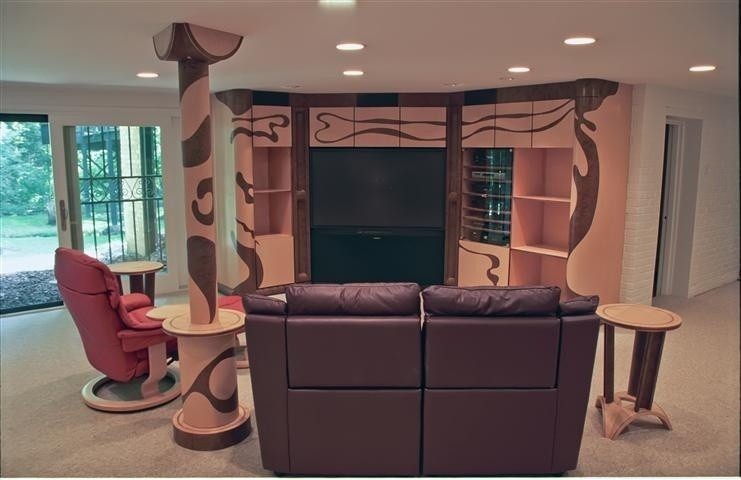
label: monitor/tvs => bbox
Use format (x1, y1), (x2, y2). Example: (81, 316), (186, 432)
(309, 147), (446, 230)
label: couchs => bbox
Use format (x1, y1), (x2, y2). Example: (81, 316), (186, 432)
(243, 282), (604, 477)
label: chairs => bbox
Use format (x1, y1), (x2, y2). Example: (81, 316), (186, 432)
(54, 247), (182, 413)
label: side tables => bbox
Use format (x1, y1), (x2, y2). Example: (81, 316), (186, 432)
(106, 259), (165, 307)
(596, 303), (683, 440)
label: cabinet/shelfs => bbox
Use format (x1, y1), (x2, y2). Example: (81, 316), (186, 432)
(208, 89), (298, 298)
(458, 78), (634, 306)
